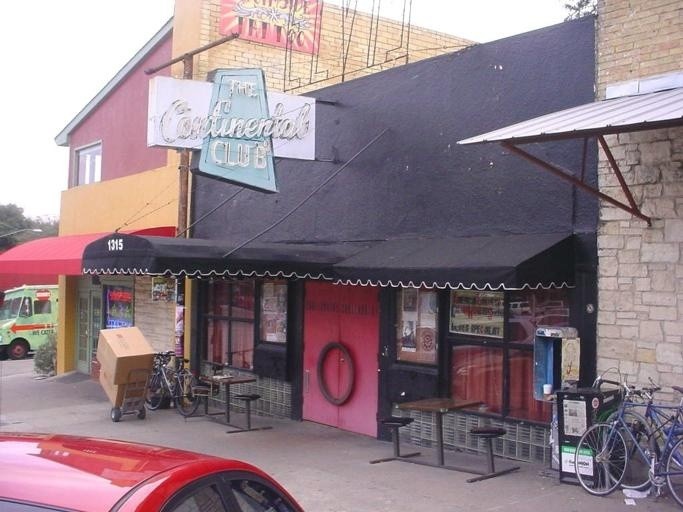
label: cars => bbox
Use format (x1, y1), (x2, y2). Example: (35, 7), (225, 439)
(0, 431), (304, 511)
(454, 323), (570, 409)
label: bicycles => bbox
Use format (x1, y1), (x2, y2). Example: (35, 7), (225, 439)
(574, 367), (683, 505)
(145, 351), (201, 416)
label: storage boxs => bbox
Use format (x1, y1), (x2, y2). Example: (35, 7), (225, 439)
(98, 365), (149, 408)
(95, 327), (156, 385)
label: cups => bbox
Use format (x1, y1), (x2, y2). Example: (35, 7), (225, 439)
(543, 383), (552, 401)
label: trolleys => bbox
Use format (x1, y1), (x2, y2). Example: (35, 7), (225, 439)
(111, 369), (152, 422)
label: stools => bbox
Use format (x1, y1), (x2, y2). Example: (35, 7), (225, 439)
(185, 386), (227, 418)
(227, 392), (273, 433)
(368, 415), (422, 463)
(467, 425), (520, 484)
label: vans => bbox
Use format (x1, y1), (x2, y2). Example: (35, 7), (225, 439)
(507, 300), (570, 347)
(0, 285), (59, 360)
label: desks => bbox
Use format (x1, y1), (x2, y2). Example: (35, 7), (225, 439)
(198, 372), (257, 430)
(395, 396), (487, 476)
